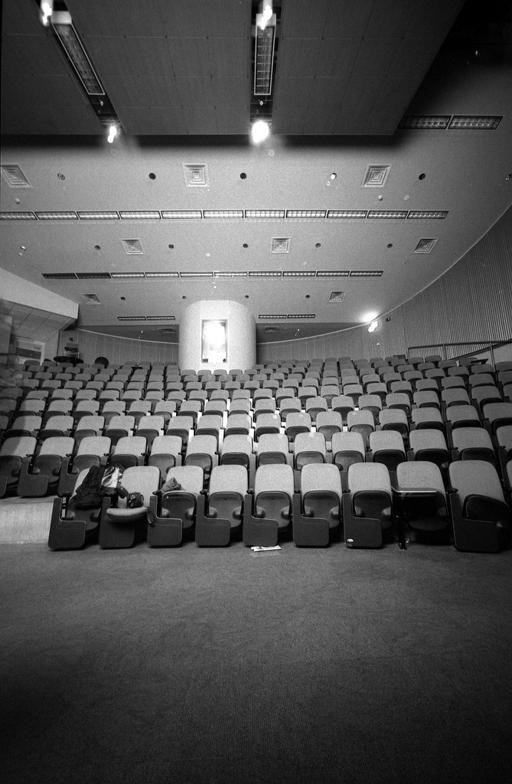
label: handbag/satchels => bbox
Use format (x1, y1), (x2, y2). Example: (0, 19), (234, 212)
(126, 493), (145, 508)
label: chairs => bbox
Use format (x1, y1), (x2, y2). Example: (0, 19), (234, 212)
(0, 355), (511, 553)
